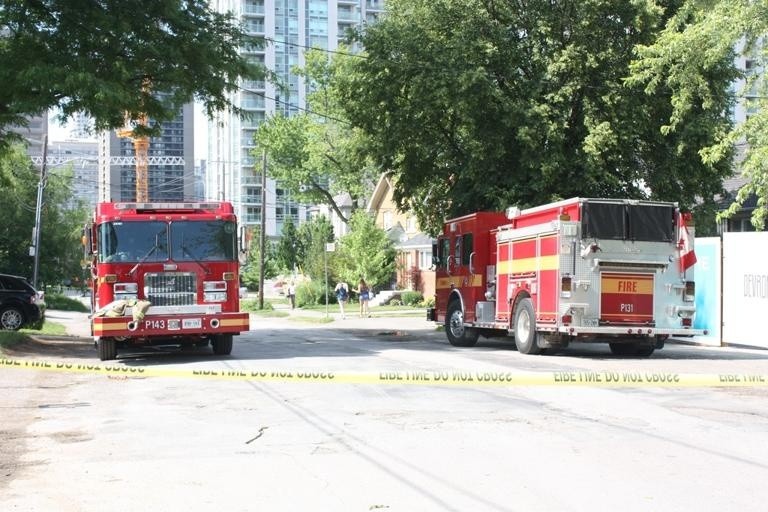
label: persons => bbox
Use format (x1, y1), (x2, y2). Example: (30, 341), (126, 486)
(351, 278), (372, 318)
(286, 280), (296, 309)
(335, 278), (350, 319)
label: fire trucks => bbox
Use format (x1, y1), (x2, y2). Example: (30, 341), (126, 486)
(79, 197), (251, 364)
(425, 192), (711, 358)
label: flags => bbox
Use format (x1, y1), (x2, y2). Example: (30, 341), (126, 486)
(677, 212), (697, 273)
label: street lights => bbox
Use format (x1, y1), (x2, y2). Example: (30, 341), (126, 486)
(32, 156), (100, 288)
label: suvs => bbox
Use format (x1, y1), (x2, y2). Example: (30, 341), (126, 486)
(0, 272), (47, 332)
